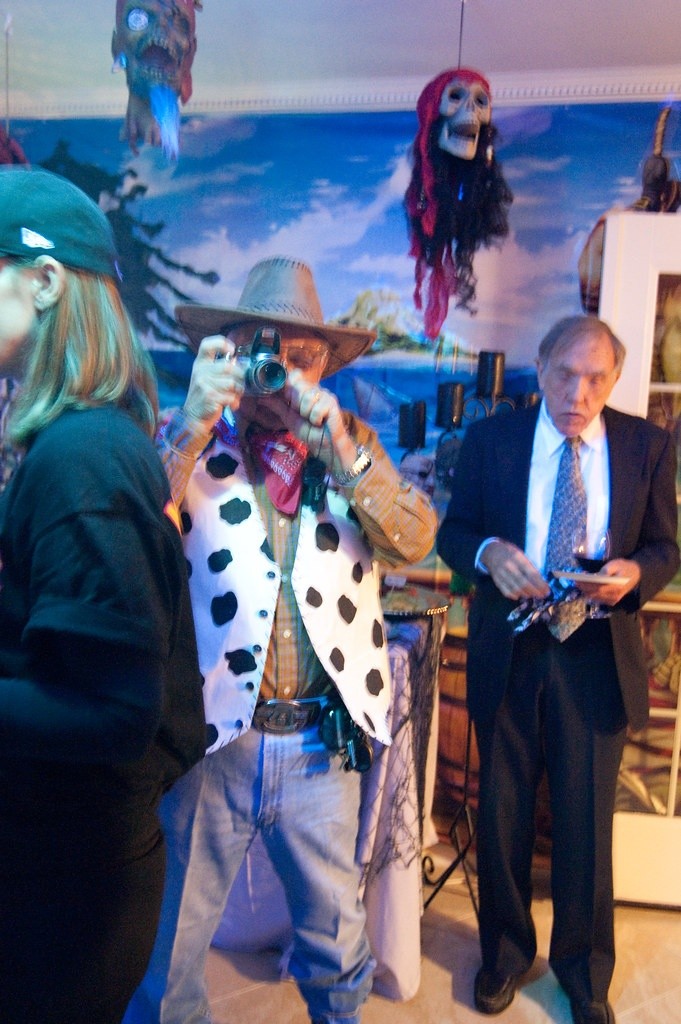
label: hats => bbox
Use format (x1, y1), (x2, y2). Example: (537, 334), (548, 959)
(173, 256), (377, 381)
(0, 166), (119, 277)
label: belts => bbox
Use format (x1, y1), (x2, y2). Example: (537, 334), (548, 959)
(252, 694), (339, 736)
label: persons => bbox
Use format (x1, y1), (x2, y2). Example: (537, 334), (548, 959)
(440, 314), (681, 1024)
(0, 167), (207, 1024)
(121, 259), (438, 1024)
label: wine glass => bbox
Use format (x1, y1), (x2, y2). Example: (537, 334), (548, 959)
(573, 526), (613, 619)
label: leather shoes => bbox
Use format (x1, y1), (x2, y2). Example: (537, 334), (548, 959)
(473, 965), (517, 1014)
(569, 996), (616, 1024)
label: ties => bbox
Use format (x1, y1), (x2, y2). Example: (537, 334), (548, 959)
(542, 438), (591, 644)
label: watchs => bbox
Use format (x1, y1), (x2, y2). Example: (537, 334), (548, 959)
(335, 446), (372, 485)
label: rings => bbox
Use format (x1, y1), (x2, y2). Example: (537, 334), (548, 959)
(312, 393), (318, 401)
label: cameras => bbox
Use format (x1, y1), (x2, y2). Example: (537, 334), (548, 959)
(214, 325), (286, 399)
